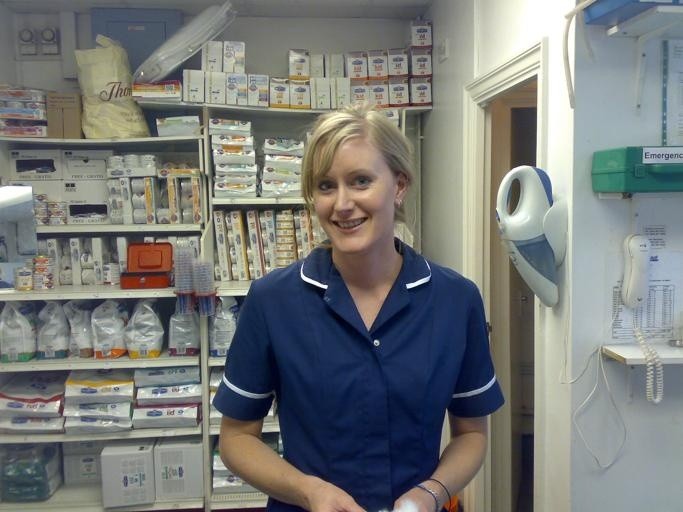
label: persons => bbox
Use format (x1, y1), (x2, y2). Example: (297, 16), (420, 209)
(211, 107), (508, 511)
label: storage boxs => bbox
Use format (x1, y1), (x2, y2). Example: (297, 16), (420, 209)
(46, 92), (83, 139)
(181, 21), (433, 110)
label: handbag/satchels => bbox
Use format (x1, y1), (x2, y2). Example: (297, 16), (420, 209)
(132, 1), (236, 83)
(74, 34), (151, 139)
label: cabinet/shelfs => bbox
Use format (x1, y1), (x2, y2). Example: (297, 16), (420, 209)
(598, 7), (682, 402)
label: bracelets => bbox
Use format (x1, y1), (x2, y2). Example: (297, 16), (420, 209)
(414, 483), (442, 512)
(423, 477), (452, 510)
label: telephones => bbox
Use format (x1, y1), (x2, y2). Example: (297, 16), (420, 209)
(622, 233), (652, 308)
